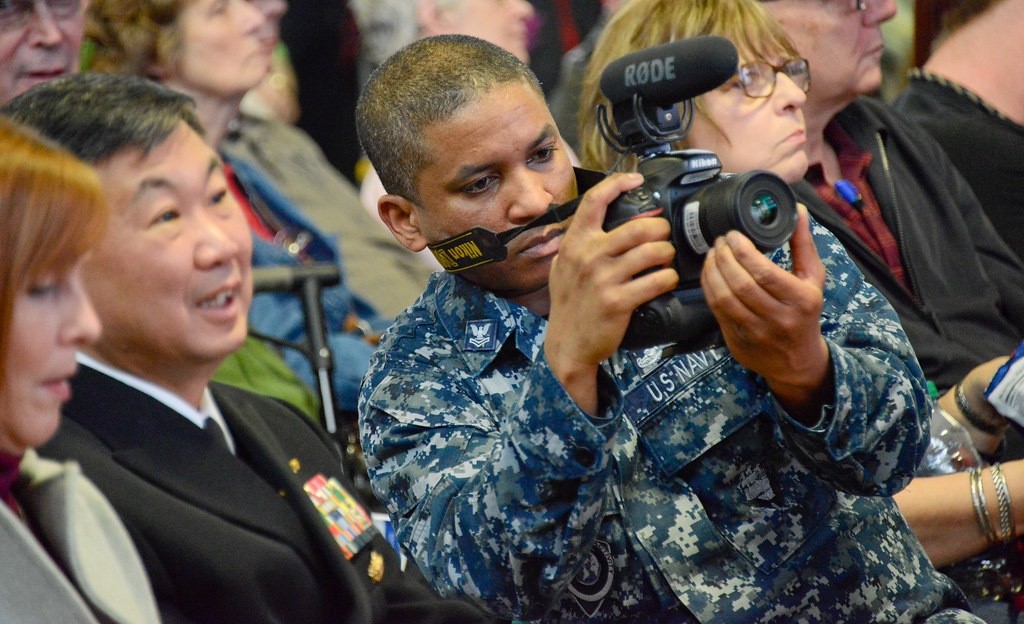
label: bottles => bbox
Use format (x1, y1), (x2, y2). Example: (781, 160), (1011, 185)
(914, 380), (983, 477)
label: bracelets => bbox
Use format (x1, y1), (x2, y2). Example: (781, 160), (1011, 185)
(970, 462), (1016, 546)
(955, 382), (1010, 436)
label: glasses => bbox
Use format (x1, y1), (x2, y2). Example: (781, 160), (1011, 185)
(717, 57), (811, 99)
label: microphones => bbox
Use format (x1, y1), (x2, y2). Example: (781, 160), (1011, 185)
(600, 36), (737, 111)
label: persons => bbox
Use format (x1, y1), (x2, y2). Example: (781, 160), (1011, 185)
(0, 0), (90, 101)
(0, 71), (490, 624)
(82, 0), (450, 419)
(0, 117), (162, 624)
(754, 0), (1024, 459)
(354, 33), (984, 624)
(582, 1), (1024, 624)
(892, 0), (1024, 264)
(360, 0), (534, 267)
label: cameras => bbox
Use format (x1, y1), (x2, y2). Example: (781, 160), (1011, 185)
(601, 147), (798, 350)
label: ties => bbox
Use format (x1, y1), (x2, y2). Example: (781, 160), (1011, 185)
(204, 418), (231, 455)
